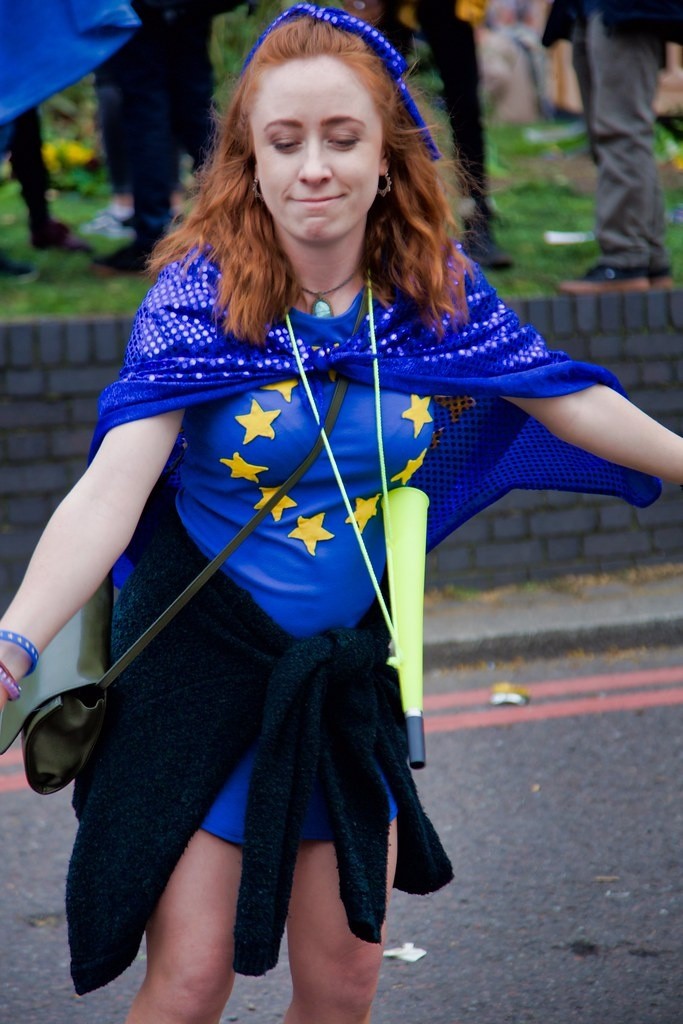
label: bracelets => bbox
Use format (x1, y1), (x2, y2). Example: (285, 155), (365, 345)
(0, 630), (38, 676)
(0, 665), (23, 701)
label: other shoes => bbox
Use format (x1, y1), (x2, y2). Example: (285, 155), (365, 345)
(78, 202), (137, 240)
(32, 226), (95, 255)
(649, 267), (674, 291)
(168, 207), (186, 232)
(0, 252), (34, 284)
(462, 216), (512, 269)
(88, 226), (166, 275)
(557, 264), (649, 293)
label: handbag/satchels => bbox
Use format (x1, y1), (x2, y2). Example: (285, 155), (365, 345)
(0, 578), (108, 796)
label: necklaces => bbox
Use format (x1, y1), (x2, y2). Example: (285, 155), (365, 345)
(301, 272), (357, 318)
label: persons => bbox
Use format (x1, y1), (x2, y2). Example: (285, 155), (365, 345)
(83, 0), (261, 271)
(542, 0), (683, 292)
(344, 1), (515, 272)
(483, 1), (585, 121)
(0, 0), (682, 1024)
(0, 0), (141, 279)
